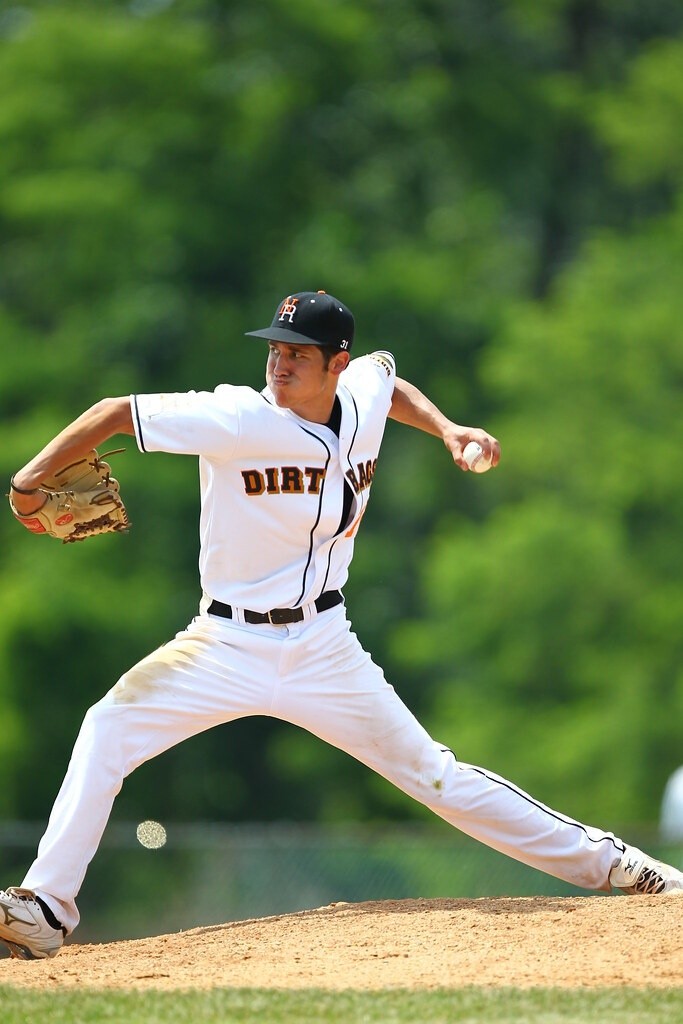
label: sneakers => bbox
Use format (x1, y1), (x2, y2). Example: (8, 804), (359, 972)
(609, 841), (683, 894)
(0, 887), (67, 960)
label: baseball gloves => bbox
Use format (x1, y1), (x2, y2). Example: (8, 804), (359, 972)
(12, 451), (129, 544)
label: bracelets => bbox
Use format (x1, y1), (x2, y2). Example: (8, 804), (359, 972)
(10, 474), (37, 495)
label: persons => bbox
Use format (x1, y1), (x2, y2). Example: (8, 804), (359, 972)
(0, 289), (683, 960)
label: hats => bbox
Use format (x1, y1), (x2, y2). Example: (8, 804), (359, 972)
(243, 289), (354, 351)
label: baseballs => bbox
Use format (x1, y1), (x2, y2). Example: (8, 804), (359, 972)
(464, 443), (494, 473)
(139, 822), (167, 849)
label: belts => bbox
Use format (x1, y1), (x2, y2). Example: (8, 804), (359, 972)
(207, 590), (343, 627)
